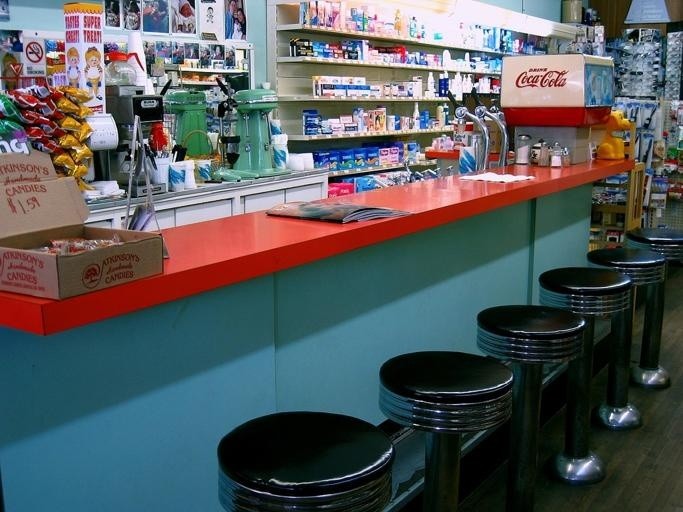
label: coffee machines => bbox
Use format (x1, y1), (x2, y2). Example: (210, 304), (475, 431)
(213, 79), (292, 183)
(163, 90), (211, 158)
(105, 79), (174, 198)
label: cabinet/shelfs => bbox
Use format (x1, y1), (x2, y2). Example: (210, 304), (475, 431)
(84, 164), (333, 233)
(590, 162), (648, 244)
(265, 0), (587, 188)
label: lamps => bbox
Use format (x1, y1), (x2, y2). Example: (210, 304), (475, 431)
(451, 1), (553, 39)
(624, 0), (672, 24)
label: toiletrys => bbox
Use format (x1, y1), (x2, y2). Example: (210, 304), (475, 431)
(421, 25), (425, 38)
(458, 21), (500, 53)
(417, 31), (421, 39)
(434, 33), (443, 40)
(298, 1), (341, 30)
(394, 10), (402, 35)
(347, 6), (394, 34)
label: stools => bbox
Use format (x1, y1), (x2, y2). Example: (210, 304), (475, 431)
(538, 267), (632, 485)
(476, 305), (586, 512)
(379, 350), (514, 511)
(217, 410), (395, 510)
(586, 246), (667, 432)
(624, 228), (682, 389)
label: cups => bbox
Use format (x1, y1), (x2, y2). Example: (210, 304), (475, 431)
(151, 158), (168, 192)
(197, 160), (211, 181)
(169, 163), (185, 192)
(273, 143), (287, 171)
(182, 160), (196, 189)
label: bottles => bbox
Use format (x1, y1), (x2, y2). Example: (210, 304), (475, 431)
(514, 134), (531, 164)
(442, 103), (449, 125)
(550, 141), (563, 168)
(563, 147), (570, 167)
(444, 72), (449, 96)
(439, 74), (444, 96)
(439, 111), (445, 126)
(428, 72), (434, 97)
(413, 102), (420, 119)
(538, 142), (550, 166)
(436, 103), (443, 120)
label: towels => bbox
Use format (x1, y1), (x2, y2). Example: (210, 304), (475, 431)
(458, 172), (538, 182)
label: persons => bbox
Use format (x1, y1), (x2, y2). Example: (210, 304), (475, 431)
(173, 0), (196, 35)
(143, 40), (250, 69)
(224, 0), (246, 41)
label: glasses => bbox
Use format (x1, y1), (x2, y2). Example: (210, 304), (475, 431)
(630, 72), (643, 75)
(665, 33), (682, 101)
(615, 49), (633, 57)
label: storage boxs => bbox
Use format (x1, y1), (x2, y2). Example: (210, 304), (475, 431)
(0, 151), (164, 300)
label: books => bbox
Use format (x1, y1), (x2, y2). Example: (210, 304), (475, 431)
(588, 231), (603, 252)
(265, 200), (410, 223)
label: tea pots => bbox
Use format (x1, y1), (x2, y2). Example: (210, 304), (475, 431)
(104, 51), (144, 86)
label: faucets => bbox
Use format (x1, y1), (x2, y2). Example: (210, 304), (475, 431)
(476, 105), (509, 168)
(454, 106), (492, 172)
